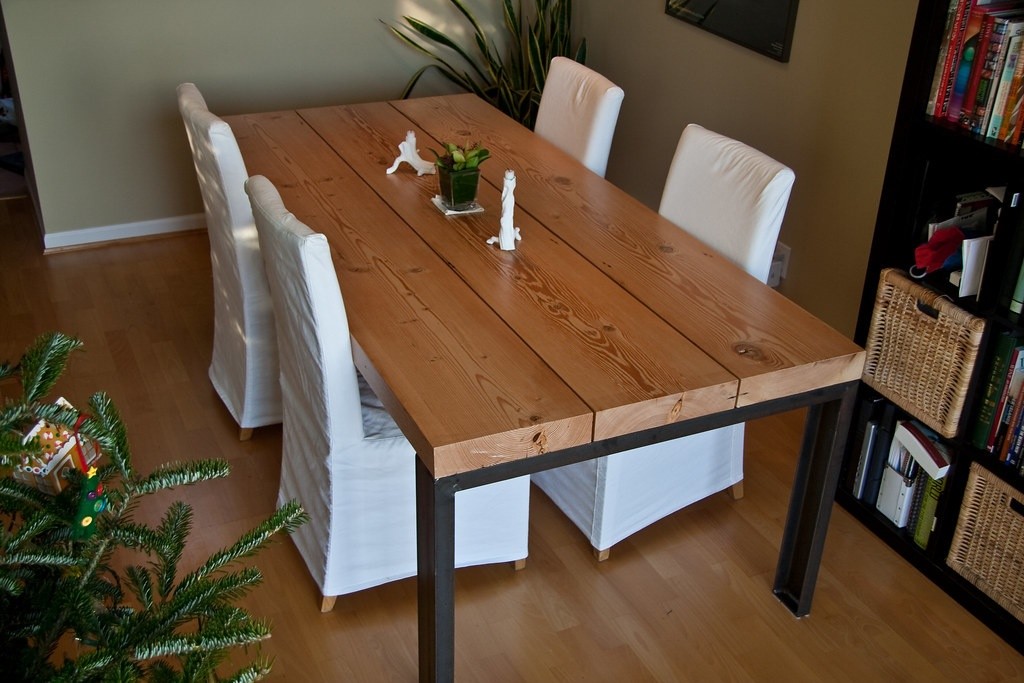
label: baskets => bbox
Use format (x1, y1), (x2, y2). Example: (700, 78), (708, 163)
(945, 462), (1024, 623)
(860, 268), (985, 438)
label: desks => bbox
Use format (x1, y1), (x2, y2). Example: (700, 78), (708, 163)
(218, 93), (866, 683)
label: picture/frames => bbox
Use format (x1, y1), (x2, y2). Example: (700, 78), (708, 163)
(665, 0), (800, 63)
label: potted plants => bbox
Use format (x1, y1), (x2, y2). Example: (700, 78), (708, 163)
(426, 138), (492, 212)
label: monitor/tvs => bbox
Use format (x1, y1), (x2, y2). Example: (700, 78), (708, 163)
(665, 0), (800, 63)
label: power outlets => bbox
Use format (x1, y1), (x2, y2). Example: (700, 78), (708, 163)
(775, 241), (792, 279)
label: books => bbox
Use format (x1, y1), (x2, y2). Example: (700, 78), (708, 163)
(1010, 261), (1024, 313)
(886, 418), (952, 550)
(926, 0), (1024, 149)
(953, 197), (993, 217)
(972, 332), (1024, 477)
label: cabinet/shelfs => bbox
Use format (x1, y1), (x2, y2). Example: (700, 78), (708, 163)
(834, 0), (1024, 655)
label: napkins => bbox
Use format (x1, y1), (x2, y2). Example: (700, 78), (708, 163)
(430, 195), (485, 216)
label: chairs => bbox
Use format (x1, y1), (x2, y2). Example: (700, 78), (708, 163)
(531, 123), (796, 563)
(534, 56), (625, 179)
(243, 173), (530, 612)
(176, 82), (283, 441)
